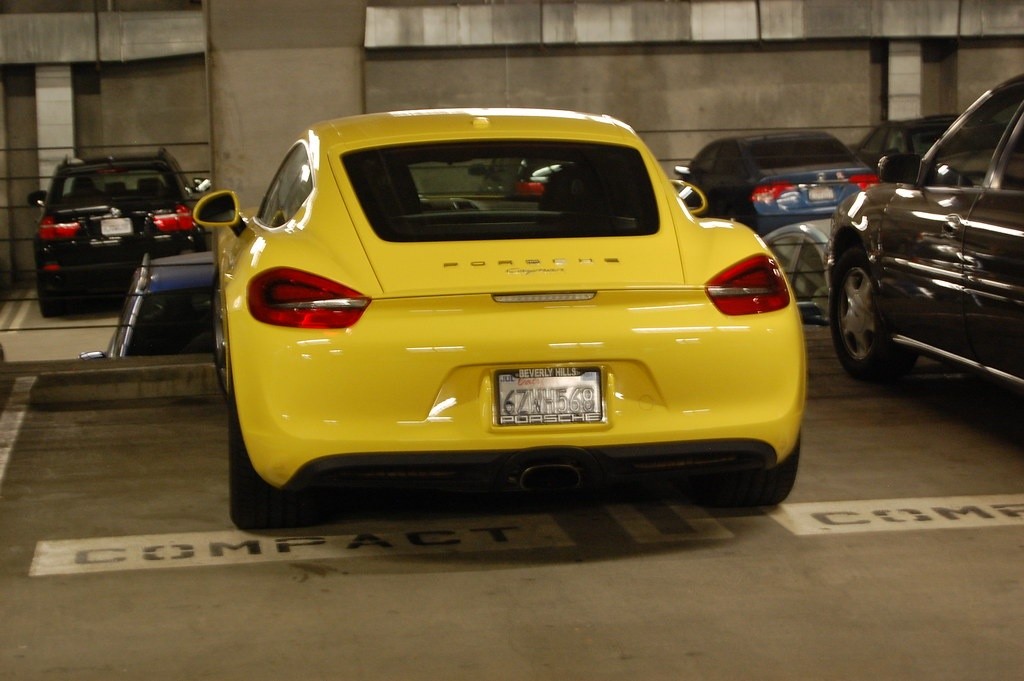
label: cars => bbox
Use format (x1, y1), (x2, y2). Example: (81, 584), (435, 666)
(673, 130), (883, 238)
(851, 115), (960, 175)
(193, 106), (806, 532)
(828, 74), (1024, 399)
(762, 219), (834, 323)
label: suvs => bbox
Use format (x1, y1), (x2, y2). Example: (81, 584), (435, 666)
(77, 251), (217, 358)
(27, 148), (210, 315)
